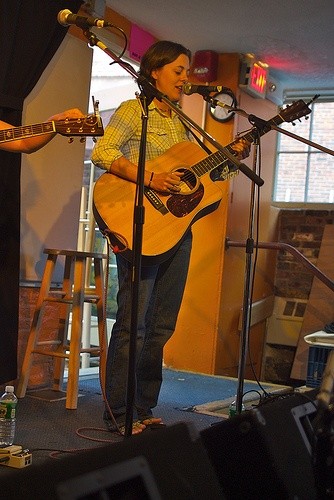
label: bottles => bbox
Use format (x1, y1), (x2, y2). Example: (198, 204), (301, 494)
(0, 386), (17, 445)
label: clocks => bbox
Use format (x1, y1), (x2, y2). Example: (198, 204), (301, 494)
(208, 88), (236, 122)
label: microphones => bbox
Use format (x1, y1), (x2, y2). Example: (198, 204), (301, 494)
(183, 83), (226, 96)
(57, 9), (112, 29)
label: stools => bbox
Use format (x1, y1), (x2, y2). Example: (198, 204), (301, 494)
(13, 248), (110, 410)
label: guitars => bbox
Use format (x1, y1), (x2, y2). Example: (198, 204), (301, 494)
(0, 96), (105, 144)
(92, 98), (313, 266)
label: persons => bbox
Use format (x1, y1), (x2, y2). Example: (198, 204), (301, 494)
(90, 40), (251, 438)
(0, 107), (86, 154)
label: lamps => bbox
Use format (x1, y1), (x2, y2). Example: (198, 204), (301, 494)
(266, 83), (277, 92)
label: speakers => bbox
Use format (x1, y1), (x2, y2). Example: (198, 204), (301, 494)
(0, 421), (226, 500)
(200, 387), (334, 500)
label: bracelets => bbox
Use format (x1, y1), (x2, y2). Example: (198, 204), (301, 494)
(147, 171), (154, 188)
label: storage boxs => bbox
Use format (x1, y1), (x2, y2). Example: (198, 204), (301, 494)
(306, 345), (334, 388)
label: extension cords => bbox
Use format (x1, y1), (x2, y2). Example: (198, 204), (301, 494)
(0, 445), (33, 469)
